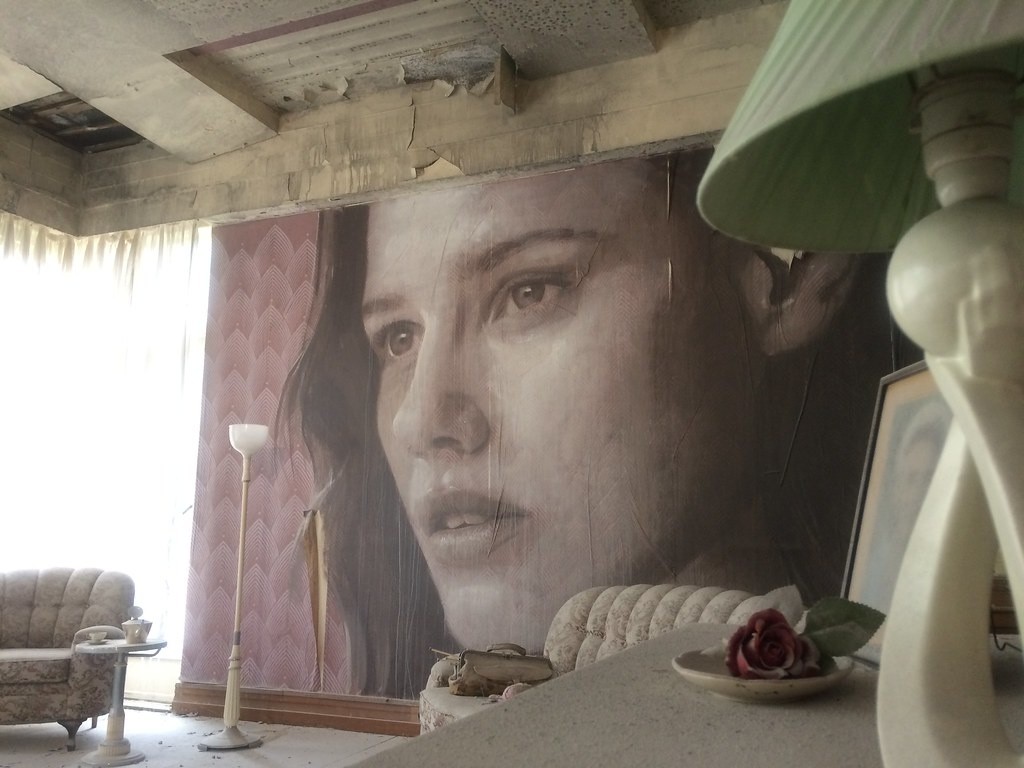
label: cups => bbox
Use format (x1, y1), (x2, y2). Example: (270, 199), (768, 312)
(86, 632), (106, 641)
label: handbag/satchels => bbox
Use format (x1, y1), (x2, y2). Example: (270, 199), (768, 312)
(448, 642), (553, 697)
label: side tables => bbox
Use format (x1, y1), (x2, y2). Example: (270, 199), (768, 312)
(74, 637), (168, 766)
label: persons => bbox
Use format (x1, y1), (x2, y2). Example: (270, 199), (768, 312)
(274, 143), (929, 702)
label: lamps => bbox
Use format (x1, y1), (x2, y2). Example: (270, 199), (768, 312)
(197, 423), (271, 751)
(693, 0), (1021, 768)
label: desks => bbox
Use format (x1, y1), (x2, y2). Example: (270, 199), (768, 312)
(344, 623), (1021, 768)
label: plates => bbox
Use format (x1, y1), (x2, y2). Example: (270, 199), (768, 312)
(87, 640), (108, 644)
(670, 646), (857, 703)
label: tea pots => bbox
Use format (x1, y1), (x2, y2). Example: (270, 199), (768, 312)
(121, 606), (154, 643)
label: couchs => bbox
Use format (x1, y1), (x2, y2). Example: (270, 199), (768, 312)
(417, 584), (763, 737)
(0, 569), (133, 750)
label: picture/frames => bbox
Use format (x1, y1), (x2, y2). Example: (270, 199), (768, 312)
(837, 359), (954, 671)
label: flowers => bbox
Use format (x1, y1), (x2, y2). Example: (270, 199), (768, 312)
(722, 596), (887, 681)
